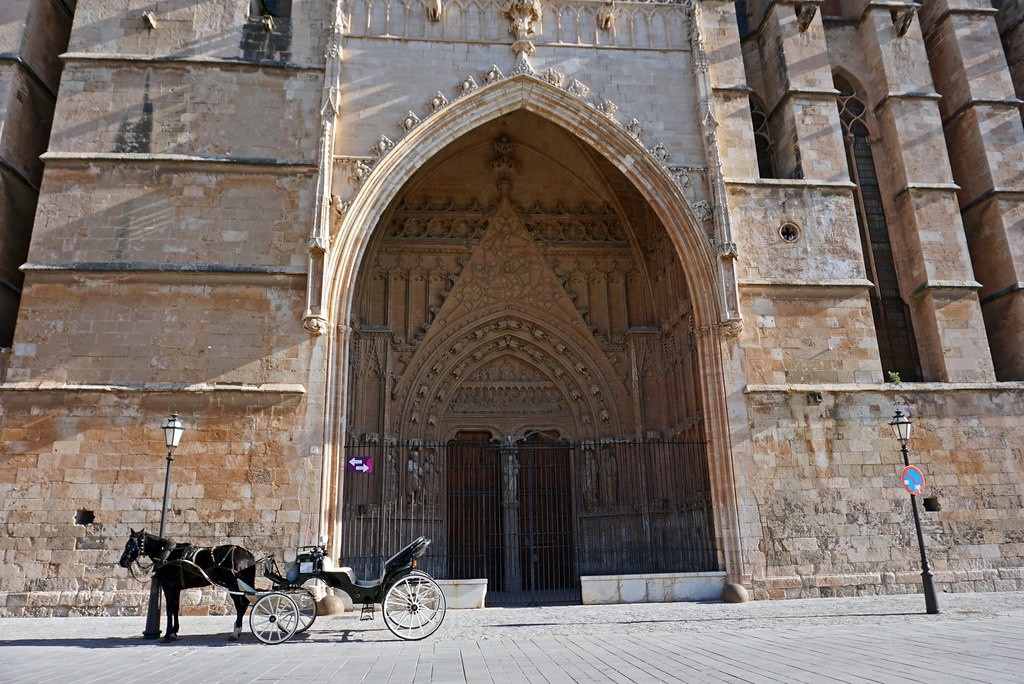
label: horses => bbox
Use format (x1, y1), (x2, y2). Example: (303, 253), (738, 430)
(121, 527), (256, 642)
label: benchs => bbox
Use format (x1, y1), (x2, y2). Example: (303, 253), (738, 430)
(380, 556), (405, 581)
(323, 556), (357, 586)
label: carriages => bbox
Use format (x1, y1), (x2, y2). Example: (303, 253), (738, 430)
(118, 527), (447, 645)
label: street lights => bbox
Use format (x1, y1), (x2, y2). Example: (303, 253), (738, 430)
(141, 410), (186, 638)
(887, 408), (941, 616)
